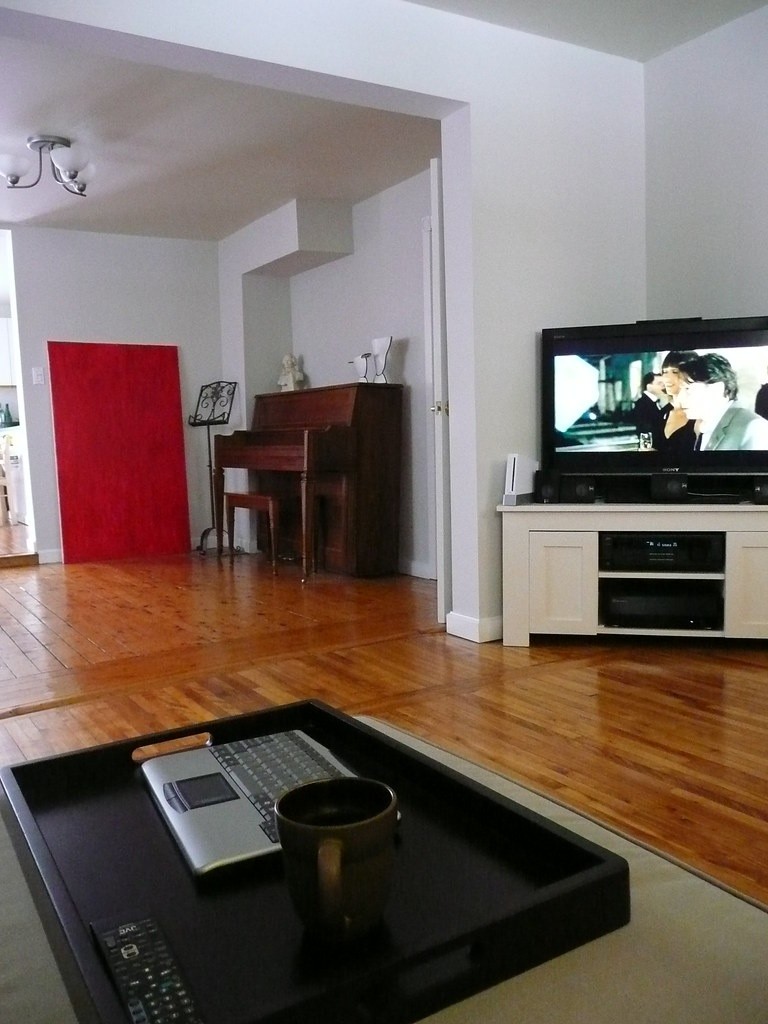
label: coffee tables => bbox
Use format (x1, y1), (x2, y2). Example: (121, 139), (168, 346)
(0, 714), (768, 1024)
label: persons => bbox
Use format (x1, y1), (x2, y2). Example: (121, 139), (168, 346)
(277, 354), (304, 386)
(634, 350), (768, 450)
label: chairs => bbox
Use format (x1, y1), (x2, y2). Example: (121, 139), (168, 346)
(0, 437), (18, 527)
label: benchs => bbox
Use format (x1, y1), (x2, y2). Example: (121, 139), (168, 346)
(220, 490), (325, 573)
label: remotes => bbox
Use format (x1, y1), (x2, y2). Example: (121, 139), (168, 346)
(90, 908), (203, 1024)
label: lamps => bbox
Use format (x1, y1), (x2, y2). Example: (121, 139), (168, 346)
(0, 135), (97, 197)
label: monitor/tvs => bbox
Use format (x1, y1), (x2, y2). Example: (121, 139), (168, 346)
(540, 316), (768, 505)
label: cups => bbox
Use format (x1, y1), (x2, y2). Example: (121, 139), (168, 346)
(640, 432), (652, 450)
(273, 777), (397, 936)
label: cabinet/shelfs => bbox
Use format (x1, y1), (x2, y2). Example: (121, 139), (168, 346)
(497, 502), (768, 648)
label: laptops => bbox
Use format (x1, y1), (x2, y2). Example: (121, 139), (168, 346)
(142, 728), (401, 876)
